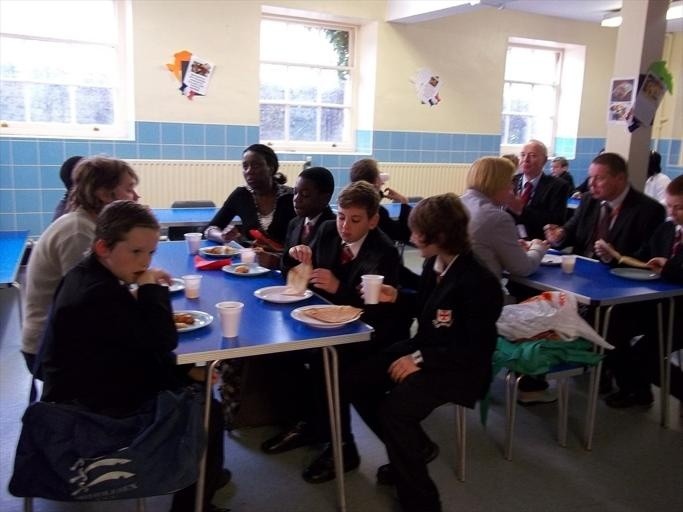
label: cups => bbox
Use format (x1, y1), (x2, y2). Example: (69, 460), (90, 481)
(561, 255), (578, 273)
(361, 274), (384, 305)
(182, 275), (203, 299)
(184, 233), (203, 255)
(215, 301), (244, 338)
(380, 173), (391, 184)
(240, 248), (256, 263)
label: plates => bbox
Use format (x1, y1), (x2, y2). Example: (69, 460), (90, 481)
(540, 253), (563, 266)
(172, 310), (213, 332)
(199, 245), (241, 258)
(157, 278), (186, 292)
(253, 285), (313, 304)
(222, 262), (270, 276)
(291, 304), (361, 328)
(610, 267), (661, 281)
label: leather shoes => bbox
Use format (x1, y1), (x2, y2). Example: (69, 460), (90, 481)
(215, 468), (231, 491)
(602, 388), (655, 410)
(302, 438), (360, 484)
(260, 424), (322, 455)
(205, 505), (232, 512)
(398, 477), (442, 512)
(377, 439), (440, 483)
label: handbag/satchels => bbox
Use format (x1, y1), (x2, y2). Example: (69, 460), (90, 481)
(6, 377), (208, 504)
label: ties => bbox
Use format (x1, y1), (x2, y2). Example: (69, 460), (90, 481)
(339, 243), (356, 266)
(669, 230), (682, 260)
(300, 222), (314, 243)
(520, 182), (532, 212)
(591, 203), (611, 253)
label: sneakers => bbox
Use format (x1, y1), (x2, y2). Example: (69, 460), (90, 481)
(516, 387), (559, 406)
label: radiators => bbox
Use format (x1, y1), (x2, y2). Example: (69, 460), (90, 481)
(120, 158), (304, 209)
(378, 162), (469, 208)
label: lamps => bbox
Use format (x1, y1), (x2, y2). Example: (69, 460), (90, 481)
(599, 0), (683, 28)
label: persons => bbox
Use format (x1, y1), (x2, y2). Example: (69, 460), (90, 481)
(350, 192), (504, 511)
(30, 198), (232, 512)
(572, 148), (603, 198)
(18, 154), (139, 381)
(258, 181), (403, 484)
(502, 143), (569, 241)
(50, 154), (84, 221)
(459, 156), (557, 403)
(350, 160), (414, 246)
(644, 152), (672, 206)
(551, 156), (575, 189)
(543, 154), (664, 393)
(249, 167), (335, 268)
(594, 176), (681, 408)
(202, 143), (292, 247)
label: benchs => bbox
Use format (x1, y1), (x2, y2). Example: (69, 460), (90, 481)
(503, 358), (593, 461)
(0, 233), (35, 328)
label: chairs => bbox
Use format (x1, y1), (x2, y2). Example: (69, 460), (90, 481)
(167, 198), (215, 241)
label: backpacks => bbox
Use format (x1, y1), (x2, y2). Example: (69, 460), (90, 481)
(488, 333), (601, 379)
